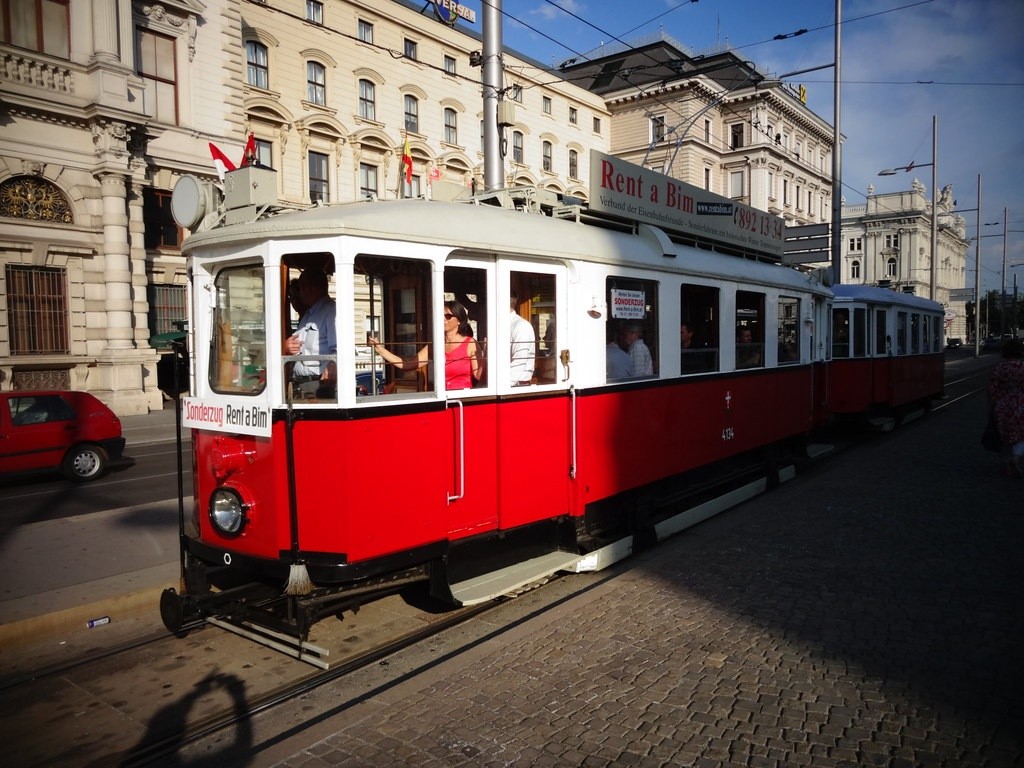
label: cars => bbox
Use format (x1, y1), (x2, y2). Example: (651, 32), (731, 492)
(947, 338), (963, 349)
(970, 333), (1018, 352)
(0, 388), (125, 483)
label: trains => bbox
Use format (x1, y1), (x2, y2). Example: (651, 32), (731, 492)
(159, 147), (945, 670)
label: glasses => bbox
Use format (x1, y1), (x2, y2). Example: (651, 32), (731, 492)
(444, 313), (456, 320)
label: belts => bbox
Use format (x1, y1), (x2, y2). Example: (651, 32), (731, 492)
(291, 375), (322, 384)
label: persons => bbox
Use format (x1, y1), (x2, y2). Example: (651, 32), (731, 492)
(366, 296), (487, 390)
(507, 285), (539, 390)
(287, 274), (308, 322)
(285, 267), (339, 401)
(986, 337), (1023, 476)
(604, 305), (764, 380)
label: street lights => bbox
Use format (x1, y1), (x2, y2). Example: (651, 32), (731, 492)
(877, 115), (938, 301)
(938, 174), (979, 358)
(756, 0), (841, 285)
(972, 207), (1008, 342)
